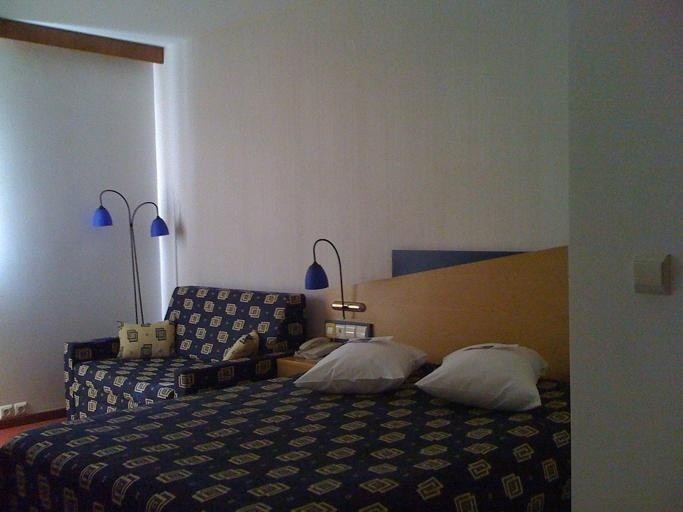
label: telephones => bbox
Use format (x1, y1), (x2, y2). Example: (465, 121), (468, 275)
(297, 336), (342, 360)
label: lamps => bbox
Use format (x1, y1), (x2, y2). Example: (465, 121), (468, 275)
(303, 238), (348, 319)
(92, 189), (170, 325)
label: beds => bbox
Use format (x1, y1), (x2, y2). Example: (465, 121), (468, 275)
(2, 246), (570, 512)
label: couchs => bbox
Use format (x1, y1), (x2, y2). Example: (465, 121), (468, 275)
(60, 283), (307, 424)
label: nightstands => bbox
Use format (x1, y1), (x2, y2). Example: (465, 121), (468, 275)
(274, 353), (318, 379)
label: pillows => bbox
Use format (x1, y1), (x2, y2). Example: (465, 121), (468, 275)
(116, 316), (178, 362)
(218, 328), (261, 363)
(294, 335), (552, 412)
